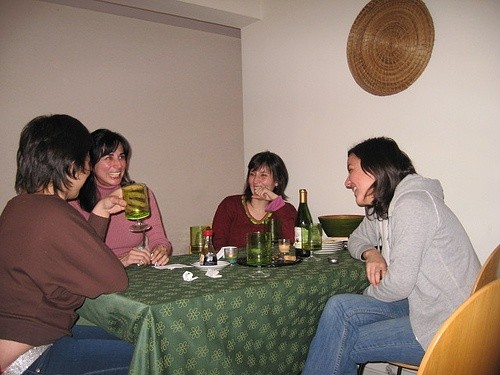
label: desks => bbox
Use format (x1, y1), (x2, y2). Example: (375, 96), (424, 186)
(75, 247), (371, 375)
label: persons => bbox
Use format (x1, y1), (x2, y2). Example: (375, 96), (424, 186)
(300, 136), (482, 375)
(212, 152), (297, 253)
(67, 129), (172, 265)
(0, 114), (145, 374)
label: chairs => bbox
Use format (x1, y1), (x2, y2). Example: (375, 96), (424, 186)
(358, 244), (500, 375)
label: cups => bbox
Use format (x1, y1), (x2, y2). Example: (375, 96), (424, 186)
(190, 226), (210, 252)
(222, 246), (238, 263)
(278, 239), (290, 255)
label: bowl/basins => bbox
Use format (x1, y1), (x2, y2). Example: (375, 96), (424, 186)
(318, 215), (365, 238)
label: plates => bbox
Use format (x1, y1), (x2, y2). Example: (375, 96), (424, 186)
(236, 254), (303, 267)
(313, 237), (348, 254)
(192, 261), (230, 271)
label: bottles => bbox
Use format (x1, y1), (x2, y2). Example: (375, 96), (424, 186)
(294, 189), (313, 257)
(200, 230), (217, 266)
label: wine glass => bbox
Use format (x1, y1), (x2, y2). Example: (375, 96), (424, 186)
(264, 218), (282, 248)
(122, 183), (151, 232)
(301, 223), (322, 262)
(246, 232), (272, 278)
